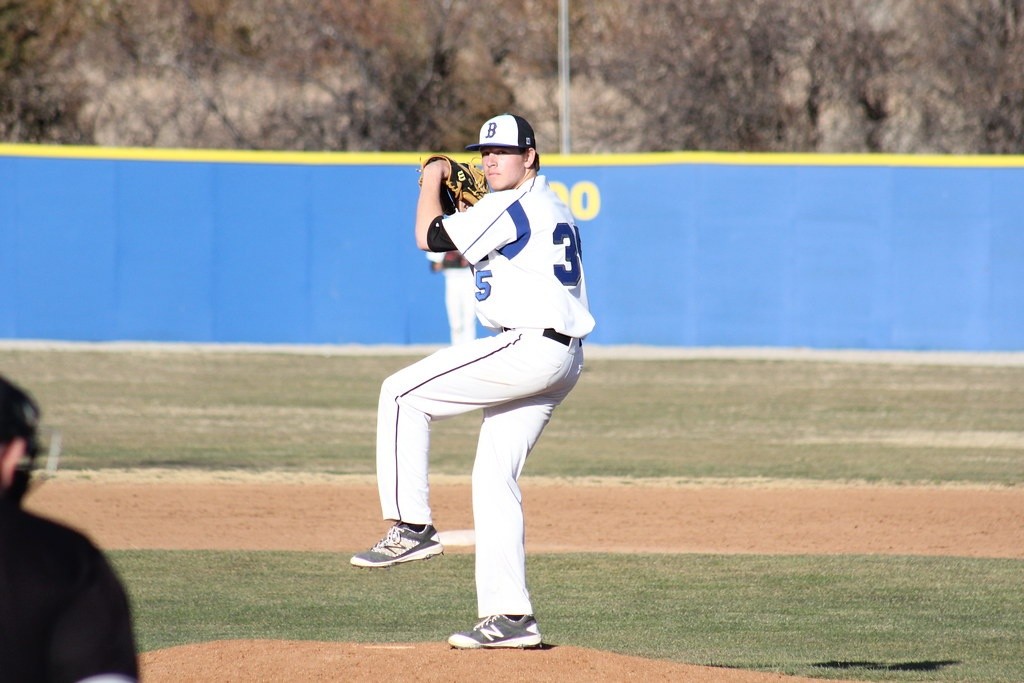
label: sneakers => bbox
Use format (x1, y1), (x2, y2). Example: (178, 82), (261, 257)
(448, 614), (541, 649)
(350, 524), (443, 568)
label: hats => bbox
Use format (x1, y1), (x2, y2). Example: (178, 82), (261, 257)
(465, 112), (536, 151)
(0, 375), (48, 457)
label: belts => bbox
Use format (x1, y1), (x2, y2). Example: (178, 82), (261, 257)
(501, 328), (583, 347)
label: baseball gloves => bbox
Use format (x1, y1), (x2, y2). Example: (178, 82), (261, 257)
(416, 155), (492, 216)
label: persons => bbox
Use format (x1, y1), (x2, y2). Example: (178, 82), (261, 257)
(350, 112), (597, 651)
(0, 374), (139, 683)
